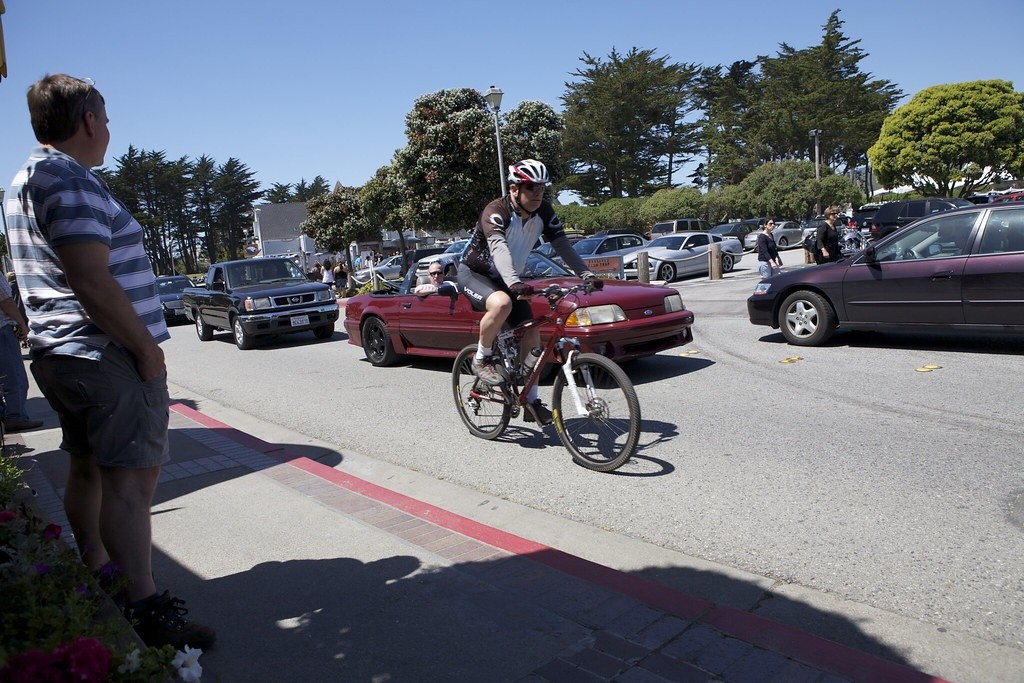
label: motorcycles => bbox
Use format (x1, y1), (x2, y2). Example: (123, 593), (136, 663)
(837, 220), (874, 260)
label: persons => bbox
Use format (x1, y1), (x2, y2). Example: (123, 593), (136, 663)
(271, 265), (281, 277)
(0, 270), (44, 433)
(311, 252), (383, 298)
(415, 262), (458, 296)
(457, 159), (605, 425)
(907, 227), (971, 259)
(758, 218), (783, 277)
(815, 206), (843, 264)
(7, 73), (217, 653)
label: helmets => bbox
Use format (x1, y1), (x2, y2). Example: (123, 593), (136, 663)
(507, 159), (549, 183)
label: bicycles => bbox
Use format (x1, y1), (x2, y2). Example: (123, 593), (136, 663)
(449, 269), (642, 475)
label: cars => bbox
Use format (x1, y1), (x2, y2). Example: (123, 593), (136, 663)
(589, 228), (652, 241)
(745, 217), (767, 228)
(154, 275), (200, 326)
(744, 220), (803, 250)
(708, 222), (750, 248)
(352, 233), (652, 289)
(620, 232), (743, 282)
(534, 234), (652, 275)
(746, 200), (1024, 348)
(802, 188), (1024, 246)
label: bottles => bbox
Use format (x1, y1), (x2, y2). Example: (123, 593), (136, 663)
(519, 346), (541, 377)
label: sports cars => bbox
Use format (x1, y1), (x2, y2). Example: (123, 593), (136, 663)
(342, 253), (696, 380)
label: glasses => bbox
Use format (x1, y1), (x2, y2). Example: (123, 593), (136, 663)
(429, 271), (444, 276)
(520, 183), (546, 191)
(829, 213), (839, 216)
(768, 222), (774, 227)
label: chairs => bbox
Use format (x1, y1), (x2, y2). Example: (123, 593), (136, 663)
(630, 239), (637, 246)
(415, 276), (431, 285)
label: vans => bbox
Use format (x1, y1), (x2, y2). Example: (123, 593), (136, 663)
(651, 217), (712, 243)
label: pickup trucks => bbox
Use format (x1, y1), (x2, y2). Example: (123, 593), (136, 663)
(182, 256), (340, 350)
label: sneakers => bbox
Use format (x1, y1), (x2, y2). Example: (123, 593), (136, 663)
(471, 353), (504, 386)
(523, 399), (553, 424)
(133, 590), (217, 651)
(4, 417), (43, 430)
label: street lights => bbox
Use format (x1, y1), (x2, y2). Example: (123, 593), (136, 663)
(808, 129), (824, 216)
(484, 85), (507, 199)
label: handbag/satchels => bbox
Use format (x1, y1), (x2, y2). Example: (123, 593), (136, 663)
(803, 226), (827, 253)
(331, 281), (337, 291)
